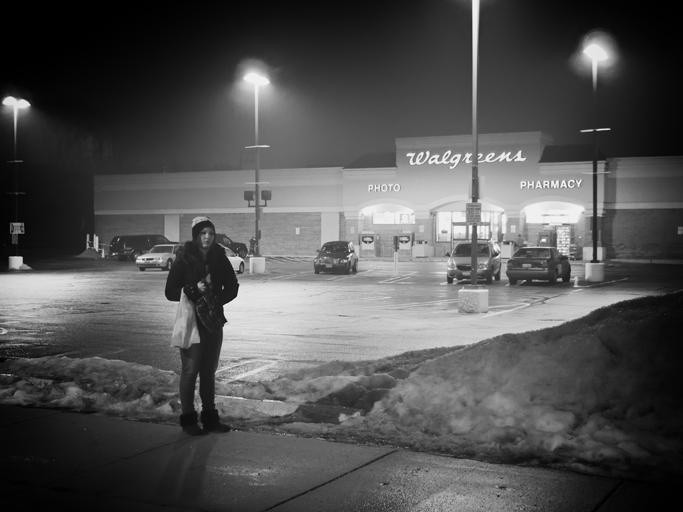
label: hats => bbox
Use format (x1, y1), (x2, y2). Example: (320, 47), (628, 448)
(191, 216), (214, 235)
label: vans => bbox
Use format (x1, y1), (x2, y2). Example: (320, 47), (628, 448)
(109, 234), (170, 263)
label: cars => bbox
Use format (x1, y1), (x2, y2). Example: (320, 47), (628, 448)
(313, 240), (359, 275)
(135, 233), (248, 273)
(446, 241), (571, 286)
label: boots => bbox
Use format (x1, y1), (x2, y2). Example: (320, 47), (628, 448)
(180, 409), (231, 435)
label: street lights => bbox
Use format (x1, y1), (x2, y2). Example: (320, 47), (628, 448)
(2, 96), (31, 272)
(580, 43), (608, 281)
(240, 71), (270, 276)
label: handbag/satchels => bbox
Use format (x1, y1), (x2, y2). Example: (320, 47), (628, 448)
(196, 295), (224, 335)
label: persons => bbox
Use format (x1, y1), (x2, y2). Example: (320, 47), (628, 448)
(165, 216), (239, 435)
(514, 234), (525, 246)
